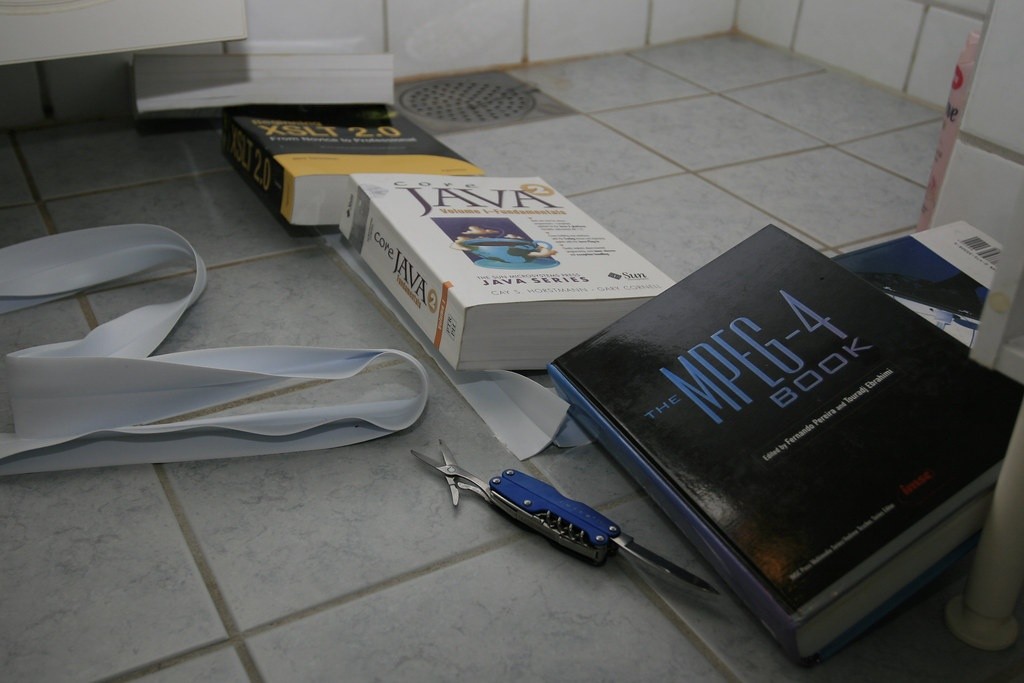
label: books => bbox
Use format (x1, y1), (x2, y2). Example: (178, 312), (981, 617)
(339, 172), (678, 372)
(827, 220), (1004, 352)
(222, 104), (484, 226)
(545, 223), (1024, 666)
(1, 0), (249, 66)
(127, 38), (395, 119)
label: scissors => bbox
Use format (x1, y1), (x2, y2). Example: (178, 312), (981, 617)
(411, 437), (492, 509)
